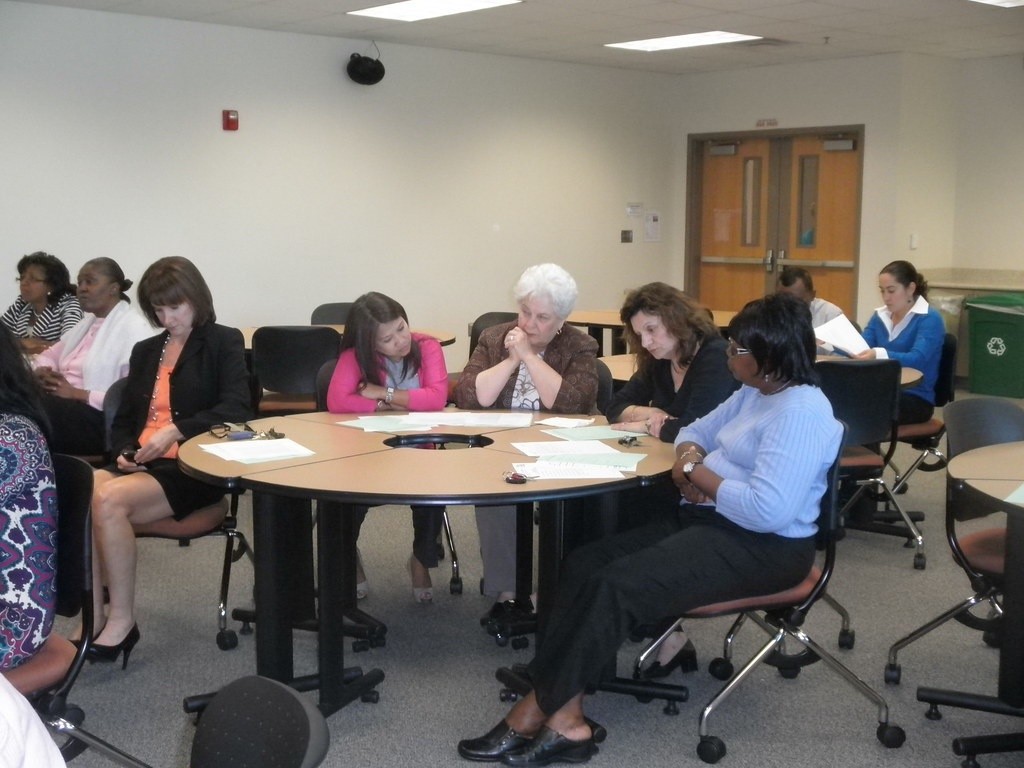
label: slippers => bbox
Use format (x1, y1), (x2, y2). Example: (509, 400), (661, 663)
(501, 725), (593, 768)
(458, 718), (534, 762)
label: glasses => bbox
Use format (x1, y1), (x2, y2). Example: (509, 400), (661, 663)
(15, 277), (46, 285)
(209, 423), (258, 439)
(728, 336), (752, 355)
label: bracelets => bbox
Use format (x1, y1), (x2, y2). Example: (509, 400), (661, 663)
(643, 419), (652, 434)
(375, 399), (381, 407)
(629, 405), (637, 422)
(680, 451), (703, 459)
(657, 415), (679, 433)
(384, 387), (394, 403)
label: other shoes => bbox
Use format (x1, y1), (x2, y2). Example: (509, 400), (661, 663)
(479, 598), (516, 625)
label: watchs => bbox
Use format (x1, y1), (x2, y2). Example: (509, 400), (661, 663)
(682, 460), (703, 473)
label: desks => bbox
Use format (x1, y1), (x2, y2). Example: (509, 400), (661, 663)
(598, 352), (641, 380)
(564, 308), (740, 333)
(917, 441), (1024, 767)
(233, 324), (455, 356)
(176, 410), (690, 722)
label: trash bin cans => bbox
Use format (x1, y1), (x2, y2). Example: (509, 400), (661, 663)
(962, 293), (1024, 399)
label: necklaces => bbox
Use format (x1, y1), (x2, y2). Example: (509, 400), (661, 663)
(452, 263), (603, 625)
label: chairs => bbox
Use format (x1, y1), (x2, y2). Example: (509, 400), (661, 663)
(52, 289), (1023, 768)
(248, 303), (356, 422)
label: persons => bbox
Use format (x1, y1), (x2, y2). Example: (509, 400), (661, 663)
(777, 268), (843, 354)
(458, 293), (843, 767)
(326, 291), (448, 605)
(66, 256), (251, 669)
(0, 250), (84, 355)
(815, 260), (946, 425)
(30, 257), (153, 457)
(603, 281), (742, 680)
(0, 320), (69, 768)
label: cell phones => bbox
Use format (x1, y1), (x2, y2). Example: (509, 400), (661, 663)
(122, 450), (153, 469)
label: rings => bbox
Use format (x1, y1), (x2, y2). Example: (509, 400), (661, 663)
(620, 422), (625, 425)
(510, 335), (514, 340)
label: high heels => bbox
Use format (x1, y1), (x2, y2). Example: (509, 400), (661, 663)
(354, 547), (369, 599)
(67, 619), (107, 658)
(643, 638), (699, 677)
(406, 557), (433, 603)
(87, 622), (141, 670)
